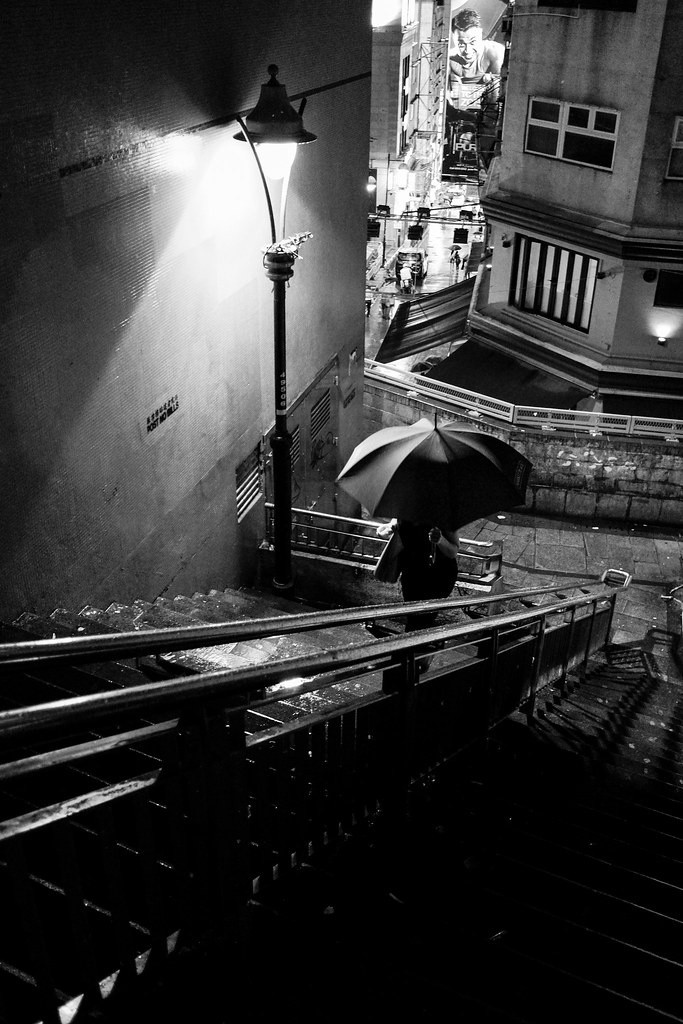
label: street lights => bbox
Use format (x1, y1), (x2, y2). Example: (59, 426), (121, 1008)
(233, 64), (318, 595)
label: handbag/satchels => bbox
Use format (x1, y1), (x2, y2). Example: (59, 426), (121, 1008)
(373, 534), (404, 584)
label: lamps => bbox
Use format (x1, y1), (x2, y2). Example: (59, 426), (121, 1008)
(368, 169), (377, 191)
(658, 338), (667, 345)
(591, 385), (600, 400)
(501, 232), (510, 248)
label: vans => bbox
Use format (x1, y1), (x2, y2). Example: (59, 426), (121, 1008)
(396, 247), (428, 281)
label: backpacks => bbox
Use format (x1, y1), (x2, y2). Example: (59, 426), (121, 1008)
(449, 257), (453, 263)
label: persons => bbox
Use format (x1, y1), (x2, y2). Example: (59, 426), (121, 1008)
(376, 517), (460, 675)
(365, 286), (372, 317)
(381, 293), (395, 318)
(450, 250), (454, 263)
(455, 251), (460, 269)
(449, 9), (505, 83)
(400, 263), (412, 290)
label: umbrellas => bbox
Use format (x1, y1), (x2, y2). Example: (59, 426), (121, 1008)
(335, 407), (533, 565)
(410, 361), (432, 372)
(448, 245), (461, 253)
(425, 357), (444, 365)
(380, 286), (399, 294)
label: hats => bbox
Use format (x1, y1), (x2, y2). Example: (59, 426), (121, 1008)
(365, 286), (372, 289)
(403, 263), (408, 267)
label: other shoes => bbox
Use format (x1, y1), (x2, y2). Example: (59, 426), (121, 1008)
(367, 314), (371, 317)
